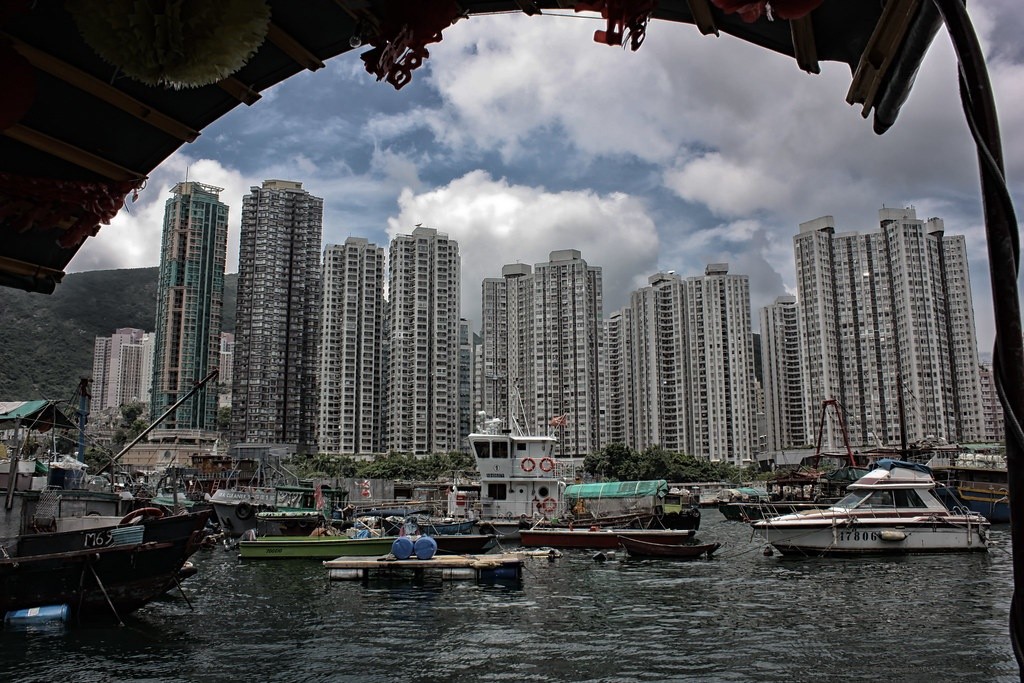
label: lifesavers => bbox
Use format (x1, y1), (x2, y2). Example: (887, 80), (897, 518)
(235, 501), (252, 521)
(540, 458), (554, 472)
(120, 507), (165, 525)
(478, 522), (497, 535)
(518, 519), (533, 530)
(543, 498), (557, 512)
(520, 457), (536, 472)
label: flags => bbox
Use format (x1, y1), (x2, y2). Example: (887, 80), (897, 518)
(548, 414), (567, 426)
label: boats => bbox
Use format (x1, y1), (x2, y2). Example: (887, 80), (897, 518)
(0, 508), (212, 624)
(518, 523), (696, 548)
(323, 553), (525, 581)
(255, 479), (350, 536)
(551, 479), (701, 530)
(239, 532), (410, 557)
(346, 506), (504, 553)
(617, 534), (721, 557)
(208, 386), (566, 539)
(714, 451), (869, 520)
(750, 459), (991, 557)
(379, 517), (480, 534)
(925, 452), (1010, 523)
